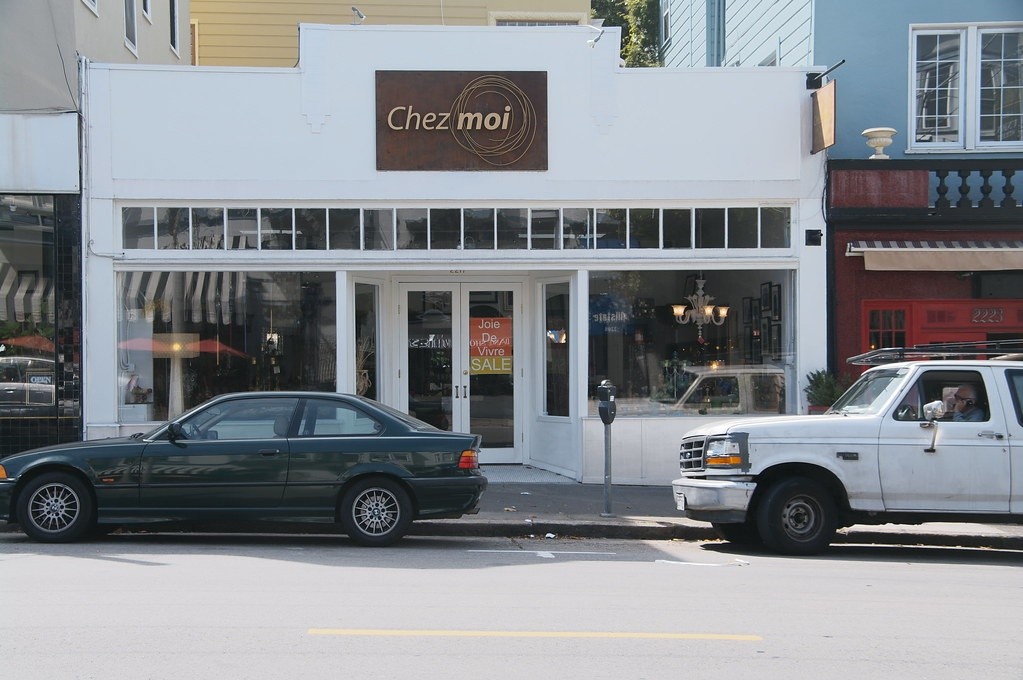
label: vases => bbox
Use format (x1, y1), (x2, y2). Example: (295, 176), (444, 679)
(860, 127), (897, 159)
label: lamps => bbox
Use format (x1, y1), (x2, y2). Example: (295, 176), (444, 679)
(351, 5), (367, 25)
(670, 271), (730, 338)
(586, 24), (605, 48)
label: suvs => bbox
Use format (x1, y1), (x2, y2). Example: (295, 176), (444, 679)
(671, 338), (1023, 559)
(587, 363), (786, 416)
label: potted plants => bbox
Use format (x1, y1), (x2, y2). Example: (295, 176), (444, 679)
(803, 370), (854, 414)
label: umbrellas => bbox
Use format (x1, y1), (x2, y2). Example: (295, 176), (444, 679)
(0, 334), (247, 359)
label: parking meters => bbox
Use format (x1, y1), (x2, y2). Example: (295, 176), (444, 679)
(596, 379), (618, 518)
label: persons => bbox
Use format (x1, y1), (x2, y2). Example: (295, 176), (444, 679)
(952, 383), (984, 422)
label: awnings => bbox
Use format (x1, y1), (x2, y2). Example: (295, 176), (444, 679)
(845, 240), (1023, 271)
(0, 235), (247, 326)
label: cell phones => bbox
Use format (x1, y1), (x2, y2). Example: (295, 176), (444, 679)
(967, 400), (974, 407)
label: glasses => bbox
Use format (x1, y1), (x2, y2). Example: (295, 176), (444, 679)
(954, 394), (972, 401)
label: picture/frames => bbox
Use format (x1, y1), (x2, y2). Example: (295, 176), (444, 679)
(683, 275), (696, 305)
(422, 291), (451, 315)
(771, 284), (781, 321)
(742, 296), (751, 324)
(760, 281), (771, 311)
(770, 325), (781, 360)
(470, 292), (498, 304)
(760, 317), (771, 356)
(751, 299), (761, 339)
(751, 337), (762, 364)
(503, 292), (513, 310)
(743, 326), (751, 359)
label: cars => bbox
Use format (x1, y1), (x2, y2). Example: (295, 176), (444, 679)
(0, 354), (79, 417)
(1, 388), (491, 547)
